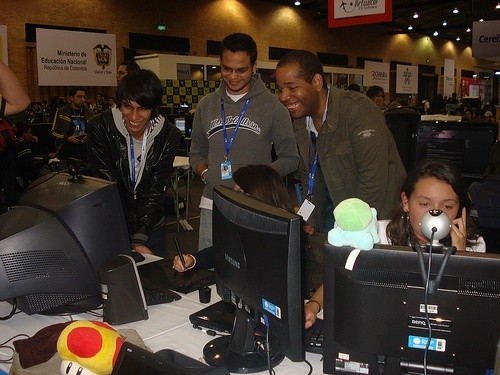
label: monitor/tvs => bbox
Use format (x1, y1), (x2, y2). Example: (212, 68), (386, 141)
(414, 121), (498, 184)
(324, 241), (499, 375)
(203, 184), (304, 374)
(112, 342), (203, 375)
(0, 171), (131, 316)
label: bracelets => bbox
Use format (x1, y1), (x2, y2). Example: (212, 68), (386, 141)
(201, 168), (208, 176)
(306, 300), (321, 313)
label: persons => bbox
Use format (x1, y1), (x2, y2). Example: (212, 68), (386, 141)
(276, 50), (407, 234)
(189, 32), (300, 250)
(87, 68), (187, 255)
(304, 156), (486, 328)
(47, 92), (115, 120)
(224, 165), (228, 171)
(13, 121), (38, 167)
(0, 54), (30, 116)
(347, 84), (497, 121)
(50, 86), (93, 159)
(172, 164), (311, 298)
(117, 62), (140, 83)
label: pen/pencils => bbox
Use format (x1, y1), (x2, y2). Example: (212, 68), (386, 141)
(172, 233), (187, 269)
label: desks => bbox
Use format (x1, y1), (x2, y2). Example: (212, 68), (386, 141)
(172, 156), (190, 232)
(0, 254), (324, 375)
(421, 115), (462, 123)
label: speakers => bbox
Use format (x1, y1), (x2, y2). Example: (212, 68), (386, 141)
(99, 253), (149, 326)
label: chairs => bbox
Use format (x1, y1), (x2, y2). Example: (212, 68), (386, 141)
(385, 107), (418, 171)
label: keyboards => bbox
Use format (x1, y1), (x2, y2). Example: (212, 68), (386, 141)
(302, 318), (324, 353)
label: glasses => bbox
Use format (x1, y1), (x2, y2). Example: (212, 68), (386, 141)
(118, 70), (128, 75)
(221, 61), (255, 74)
